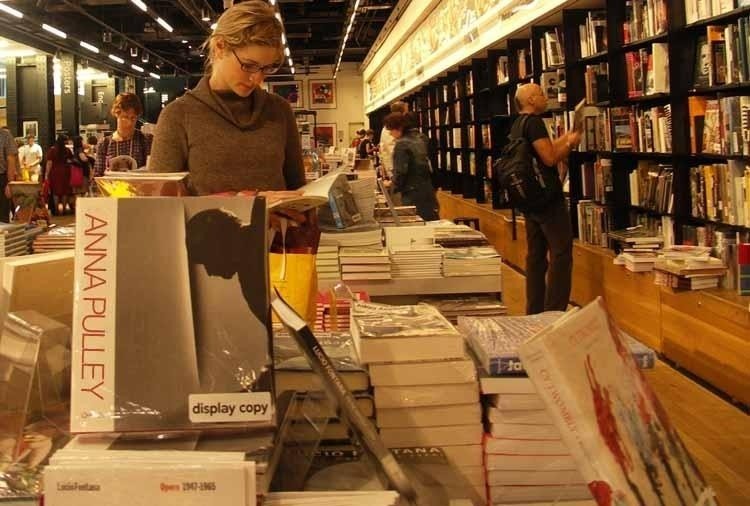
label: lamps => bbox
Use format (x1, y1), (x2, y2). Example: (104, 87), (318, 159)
(200, 2), (218, 30)
(51, 43), (130, 81)
(101, 25), (168, 69)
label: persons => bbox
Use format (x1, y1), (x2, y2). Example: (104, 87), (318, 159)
(352, 102), (440, 223)
(148, 0), (306, 253)
(510, 82), (581, 313)
(2, 93), (148, 220)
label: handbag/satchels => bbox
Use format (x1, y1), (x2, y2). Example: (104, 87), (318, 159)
(493, 113), (558, 212)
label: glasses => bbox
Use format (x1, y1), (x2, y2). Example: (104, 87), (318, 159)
(230, 49), (280, 76)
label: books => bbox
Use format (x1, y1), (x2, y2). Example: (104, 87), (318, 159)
(411, 1), (750, 290)
(2, 172), (502, 506)
(458, 296), (719, 505)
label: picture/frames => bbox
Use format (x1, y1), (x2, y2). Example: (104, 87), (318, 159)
(268, 77), (363, 167)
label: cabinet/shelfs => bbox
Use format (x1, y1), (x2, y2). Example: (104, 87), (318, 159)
(358, 0), (750, 413)
